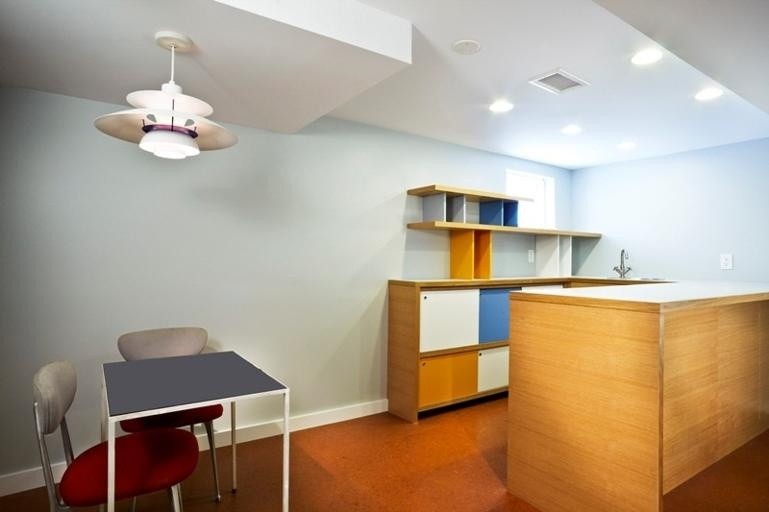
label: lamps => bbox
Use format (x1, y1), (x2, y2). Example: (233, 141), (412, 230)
(92, 32), (238, 160)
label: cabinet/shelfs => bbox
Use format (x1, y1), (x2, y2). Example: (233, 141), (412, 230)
(386, 279), (569, 425)
(401, 185), (603, 279)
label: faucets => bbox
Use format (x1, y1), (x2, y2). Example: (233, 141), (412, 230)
(621, 249), (628, 270)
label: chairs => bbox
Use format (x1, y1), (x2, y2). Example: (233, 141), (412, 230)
(116, 326), (225, 512)
(32, 359), (199, 512)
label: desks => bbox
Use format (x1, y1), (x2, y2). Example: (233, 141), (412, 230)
(98, 350), (291, 512)
